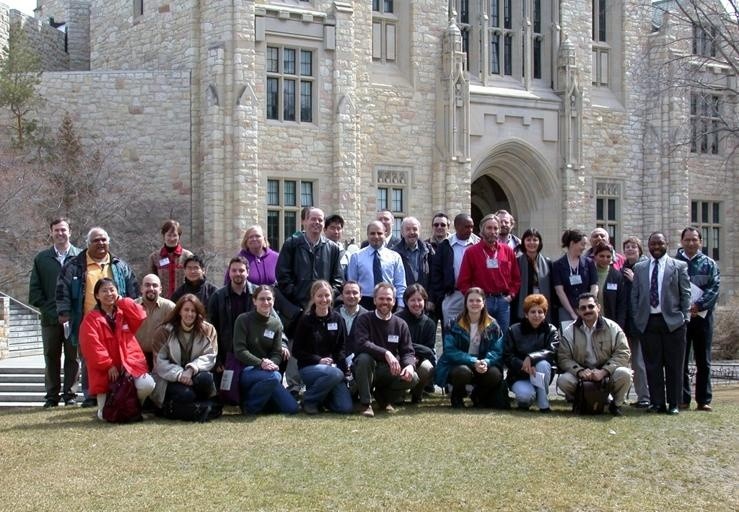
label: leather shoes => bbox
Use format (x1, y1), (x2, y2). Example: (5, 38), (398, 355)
(574, 400), (712, 416)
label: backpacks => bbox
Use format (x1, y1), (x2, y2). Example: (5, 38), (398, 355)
(103, 370), (143, 424)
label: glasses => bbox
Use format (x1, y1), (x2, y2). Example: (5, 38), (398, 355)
(434, 222), (447, 227)
(598, 255), (611, 258)
(580, 304), (595, 311)
(259, 296), (272, 301)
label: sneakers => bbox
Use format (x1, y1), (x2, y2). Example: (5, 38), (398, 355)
(301, 397), (318, 415)
(42, 398), (97, 408)
(386, 403), (394, 411)
(361, 404), (375, 416)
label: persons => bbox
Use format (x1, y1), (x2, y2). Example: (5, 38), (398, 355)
(25, 218), (85, 408)
(54, 226), (137, 409)
(349, 280), (416, 417)
(394, 284), (438, 406)
(552, 229), (599, 323)
(675, 226), (721, 411)
(150, 292), (215, 422)
(276, 207), (344, 402)
(232, 285), (298, 415)
(620, 236), (651, 402)
(629, 231), (690, 411)
(584, 226), (625, 271)
(291, 281), (352, 415)
(134, 273), (177, 407)
(75, 278), (151, 420)
(503, 292), (560, 413)
(323, 210), (521, 334)
(223, 226), (279, 287)
(147, 220), (193, 298)
(510, 229), (556, 327)
(555, 292), (634, 416)
(208, 257), (260, 419)
(594, 240), (628, 329)
(434, 288), (504, 407)
(171, 255), (220, 306)
(291, 279), (352, 415)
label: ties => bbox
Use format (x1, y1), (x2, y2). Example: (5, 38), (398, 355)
(372, 251), (385, 287)
(650, 259), (659, 308)
